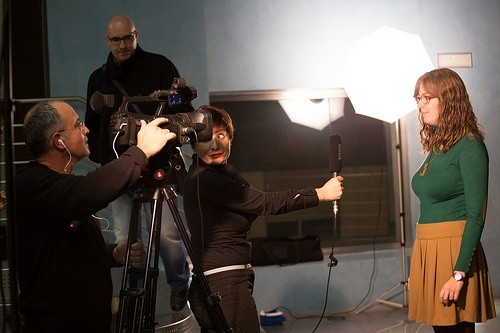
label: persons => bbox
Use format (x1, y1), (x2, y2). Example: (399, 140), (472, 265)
(408, 68), (496, 333)
(85, 15), (194, 310)
(11, 99), (177, 333)
(183, 105), (345, 333)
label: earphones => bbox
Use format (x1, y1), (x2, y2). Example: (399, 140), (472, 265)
(58, 139), (66, 147)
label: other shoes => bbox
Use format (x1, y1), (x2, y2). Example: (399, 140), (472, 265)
(169, 290), (191, 314)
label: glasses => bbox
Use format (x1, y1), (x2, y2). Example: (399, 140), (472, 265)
(108, 31), (135, 45)
(416, 95), (437, 104)
(57, 121), (84, 134)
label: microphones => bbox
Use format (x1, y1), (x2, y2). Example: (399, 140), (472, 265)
(329, 135), (342, 214)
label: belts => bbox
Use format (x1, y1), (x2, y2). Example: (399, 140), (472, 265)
(192, 264), (251, 276)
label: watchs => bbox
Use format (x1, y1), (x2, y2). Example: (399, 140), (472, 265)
(452, 272), (465, 282)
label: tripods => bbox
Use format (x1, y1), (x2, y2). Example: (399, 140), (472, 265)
(114, 155), (235, 333)
